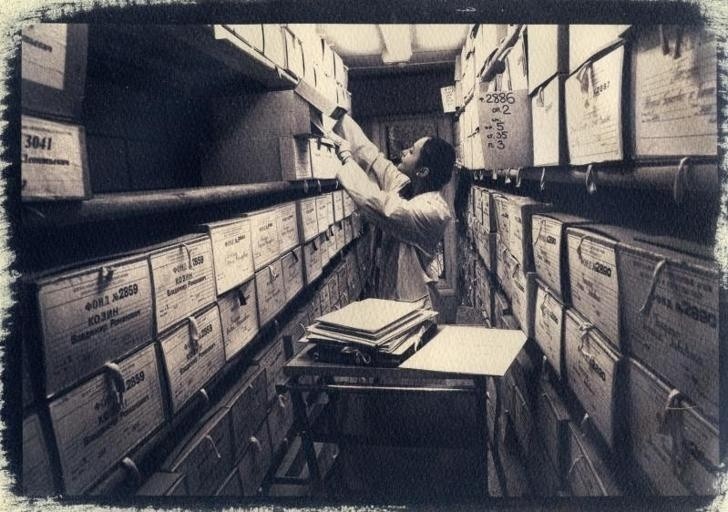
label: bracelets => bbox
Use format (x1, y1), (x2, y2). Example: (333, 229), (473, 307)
(338, 148), (351, 164)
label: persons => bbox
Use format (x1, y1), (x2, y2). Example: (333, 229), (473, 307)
(332, 112), (456, 320)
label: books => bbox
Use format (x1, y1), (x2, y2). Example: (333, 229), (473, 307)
(297, 298), (440, 367)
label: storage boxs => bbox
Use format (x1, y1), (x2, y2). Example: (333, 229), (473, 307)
(19, 113), (95, 205)
(198, 22), (357, 182)
(22, 20), (91, 122)
